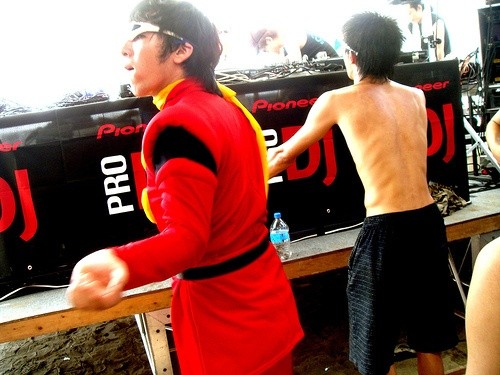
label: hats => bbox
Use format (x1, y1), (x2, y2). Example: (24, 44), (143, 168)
(249, 28), (267, 54)
(389, 0), (421, 6)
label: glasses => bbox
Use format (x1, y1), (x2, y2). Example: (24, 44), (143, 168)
(127, 20), (190, 46)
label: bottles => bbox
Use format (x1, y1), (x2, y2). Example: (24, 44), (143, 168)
(270, 212), (291, 262)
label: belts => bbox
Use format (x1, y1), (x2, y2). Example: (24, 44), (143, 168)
(169, 231), (271, 280)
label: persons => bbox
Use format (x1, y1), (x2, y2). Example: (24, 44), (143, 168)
(466, 236), (500, 375)
(390, 1), (451, 61)
(248, 27), (341, 63)
(485, 108), (500, 163)
(268, 10), (460, 375)
(64, 0), (305, 375)
